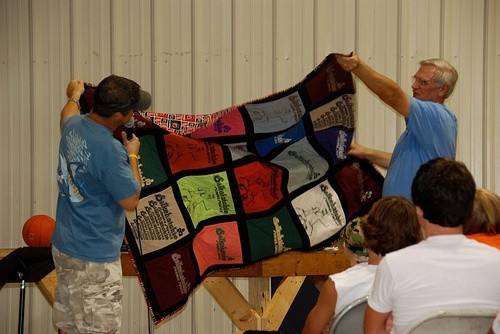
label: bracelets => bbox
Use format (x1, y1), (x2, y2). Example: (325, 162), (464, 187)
(129, 154), (138, 162)
(68, 97), (80, 108)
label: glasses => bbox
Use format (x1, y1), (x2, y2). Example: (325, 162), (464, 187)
(411, 76), (433, 88)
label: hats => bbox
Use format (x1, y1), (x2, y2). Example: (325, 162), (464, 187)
(340, 217), (370, 257)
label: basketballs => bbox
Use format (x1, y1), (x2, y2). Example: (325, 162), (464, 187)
(21, 213), (56, 249)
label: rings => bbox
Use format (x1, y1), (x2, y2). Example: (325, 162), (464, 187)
(345, 64), (346, 66)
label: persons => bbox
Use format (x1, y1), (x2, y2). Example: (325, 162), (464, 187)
(51, 74), (152, 334)
(336, 53), (458, 201)
(303, 195), (423, 334)
(339, 217), (369, 268)
(363, 157), (500, 334)
(464, 189), (500, 249)
